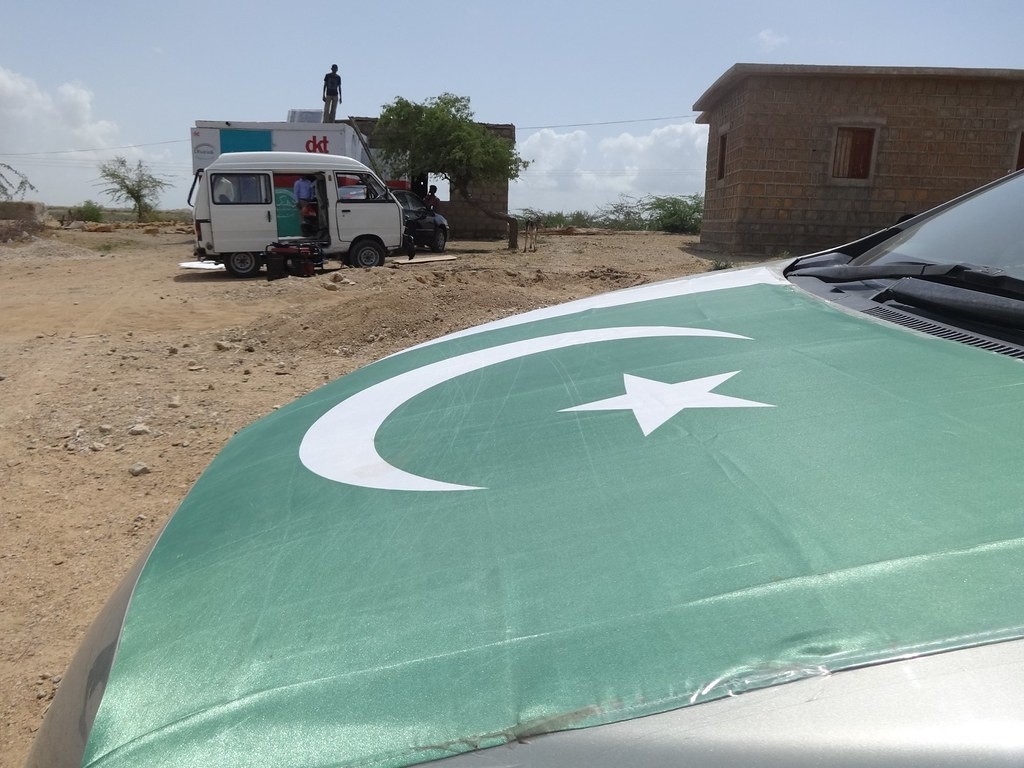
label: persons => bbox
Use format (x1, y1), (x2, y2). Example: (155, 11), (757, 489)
(212, 174), (235, 203)
(294, 174), (314, 236)
(423, 185), (440, 211)
(321, 64), (343, 123)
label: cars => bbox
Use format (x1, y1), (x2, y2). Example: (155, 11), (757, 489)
(79, 158), (1024, 767)
(338, 184), (450, 253)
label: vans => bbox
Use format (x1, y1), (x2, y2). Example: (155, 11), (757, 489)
(190, 151), (408, 279)
